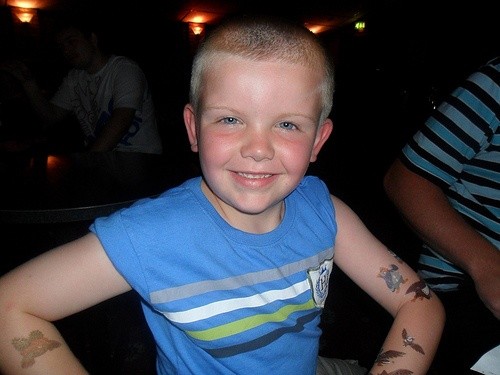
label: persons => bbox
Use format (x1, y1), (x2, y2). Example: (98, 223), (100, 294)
(0, 12), (163, 155)
(382, 53), (500, 321)
(73, 150), (150, 186)
(0, 21), (446, 375)
